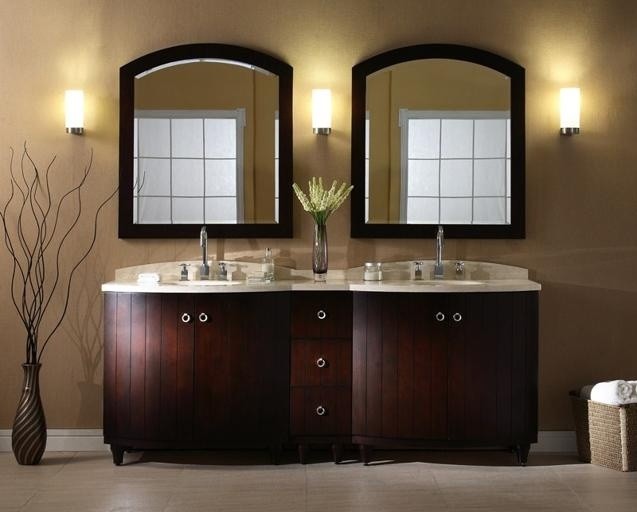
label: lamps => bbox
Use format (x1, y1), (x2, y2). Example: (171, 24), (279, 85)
(311, 88), (331, 135)
(558, 87), (582, 135)
(64, 90), (84, 134)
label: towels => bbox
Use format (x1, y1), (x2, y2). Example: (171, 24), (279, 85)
(578, 380), (636, 406)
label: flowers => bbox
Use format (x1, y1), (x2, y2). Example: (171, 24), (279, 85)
(291, 178), (355, 223)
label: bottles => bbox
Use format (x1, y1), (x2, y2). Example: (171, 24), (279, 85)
(261, 247), (274, 280)
(362, 262), (383, 281)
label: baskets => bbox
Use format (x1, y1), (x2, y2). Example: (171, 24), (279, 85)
(568, 390), (636, 472)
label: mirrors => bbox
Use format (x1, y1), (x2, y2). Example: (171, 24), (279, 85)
(120, 42), (293, 240)
(352, 43), (525, 240)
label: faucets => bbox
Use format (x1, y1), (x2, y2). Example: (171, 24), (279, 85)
(199, 226), (210, 277)
(435, 226), (444, 278)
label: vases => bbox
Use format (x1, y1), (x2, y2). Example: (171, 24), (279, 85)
(12, 363), (47, 464)
(314, 223), (328, 274)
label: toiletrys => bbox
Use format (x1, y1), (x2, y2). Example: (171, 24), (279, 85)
(263, 248), (275, 281)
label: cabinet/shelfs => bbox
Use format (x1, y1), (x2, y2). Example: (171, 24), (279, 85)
(348, 278), (542, 467)
(103, 279), (295, 466)
(292, 279), (353, 465)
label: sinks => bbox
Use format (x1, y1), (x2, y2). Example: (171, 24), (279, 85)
(410, 280), (485, 286)
(157, 280), (242, 286)
